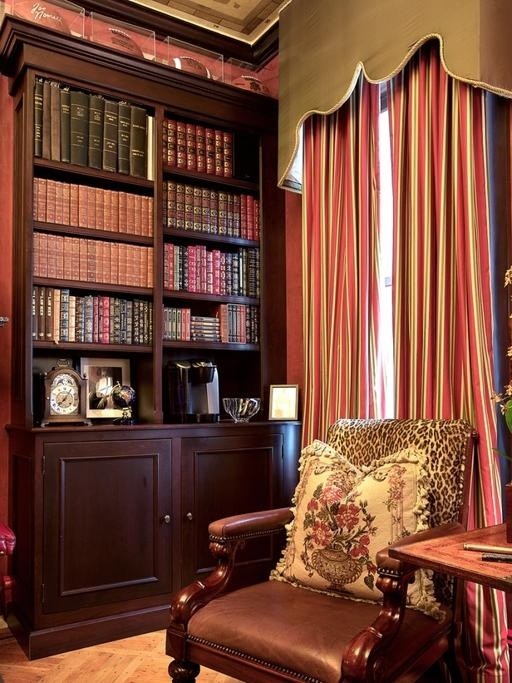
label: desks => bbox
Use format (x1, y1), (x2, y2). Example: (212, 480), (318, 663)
(391, 520), (512, 608)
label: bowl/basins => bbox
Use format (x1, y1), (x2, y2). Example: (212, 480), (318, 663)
(222, 397), (261, 424)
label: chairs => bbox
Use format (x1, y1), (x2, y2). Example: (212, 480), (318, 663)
(166, 420), (480, 683)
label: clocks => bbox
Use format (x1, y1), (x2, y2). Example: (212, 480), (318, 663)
(40, 358), (88, 427)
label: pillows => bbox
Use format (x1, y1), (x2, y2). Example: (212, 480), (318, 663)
(281, 442), (440, 617)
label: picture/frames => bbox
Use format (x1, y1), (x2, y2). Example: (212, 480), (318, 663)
(268, 383), (299, 421)
(74, 352), (137, 423)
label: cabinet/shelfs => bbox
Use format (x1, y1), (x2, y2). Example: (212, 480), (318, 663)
(2, 13), (303, 661)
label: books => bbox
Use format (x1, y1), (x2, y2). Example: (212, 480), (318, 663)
(32, 287), (153, 344)
(162, 304), (260, 344)
(164, 242), (260, 298)
(162, 180), (261, 241)
(32, 178), (155, 237)
(31, 78), (155, 181)
(162, 118), (232, 178)
(32, 232), (154, 288)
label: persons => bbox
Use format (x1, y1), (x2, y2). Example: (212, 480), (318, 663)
(96, 368), (114, 408)
